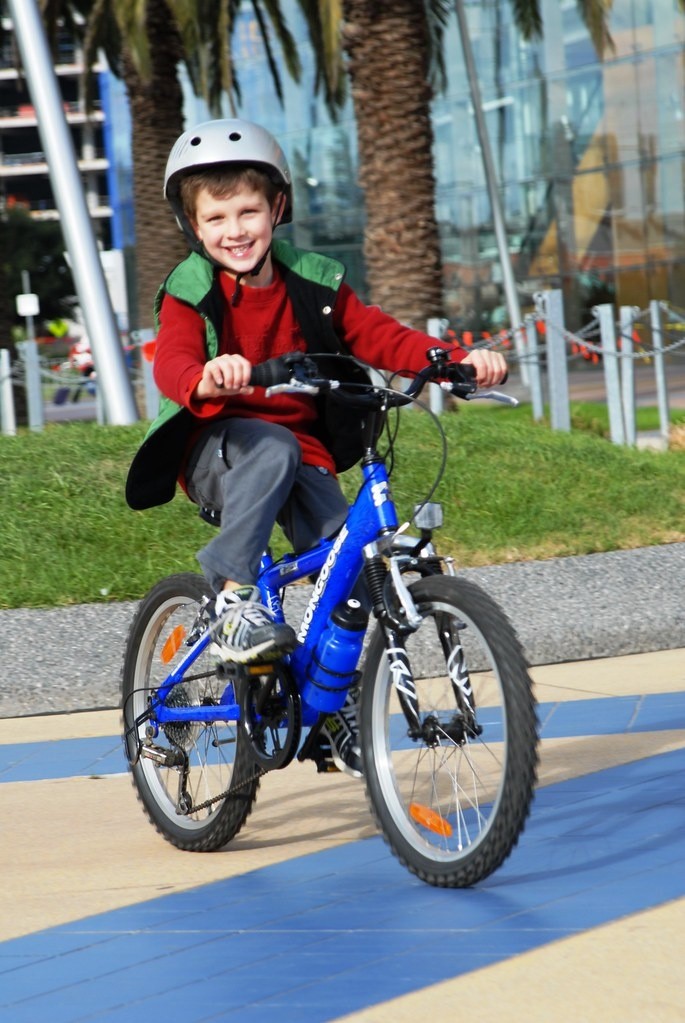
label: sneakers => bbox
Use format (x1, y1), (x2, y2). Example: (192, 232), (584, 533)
(208, 585), (297, 664)
(321, 685), (364, 779)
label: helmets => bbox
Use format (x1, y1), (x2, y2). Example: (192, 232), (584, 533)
(162, 118), (294, 253)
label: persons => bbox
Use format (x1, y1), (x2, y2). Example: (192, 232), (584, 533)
(152, 117), (508, 781)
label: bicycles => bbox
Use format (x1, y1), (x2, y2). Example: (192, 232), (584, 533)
(118, 347), (542, 888)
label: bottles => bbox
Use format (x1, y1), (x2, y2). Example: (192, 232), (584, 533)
(302, 598), (368, 712)
